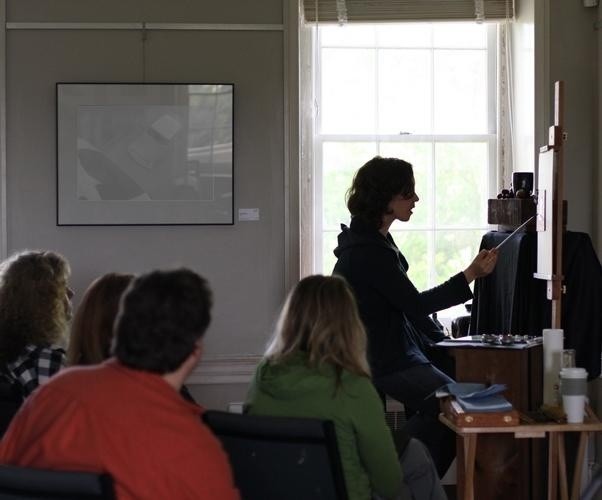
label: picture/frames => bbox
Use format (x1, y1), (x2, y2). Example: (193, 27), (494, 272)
(56, 82), (235, 226)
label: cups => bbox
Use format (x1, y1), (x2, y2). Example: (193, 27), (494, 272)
(559, 367), (588, 423)
(512, 172), (533, 195)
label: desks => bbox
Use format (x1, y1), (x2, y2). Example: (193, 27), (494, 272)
(438, 402), (602, 500)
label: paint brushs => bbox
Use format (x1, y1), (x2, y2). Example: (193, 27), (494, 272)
(496, 214), (537, 250)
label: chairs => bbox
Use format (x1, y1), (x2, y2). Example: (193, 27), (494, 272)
(0, 464), (115, 500)
(202, 409), (349, 500)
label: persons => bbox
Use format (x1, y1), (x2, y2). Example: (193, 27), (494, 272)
(0, 248), (76, 402)
(330, 155), (500, 478)
(242, 274), (449, 500)
(65, 270), (140, 366)
(0, 266), (242, 500)
